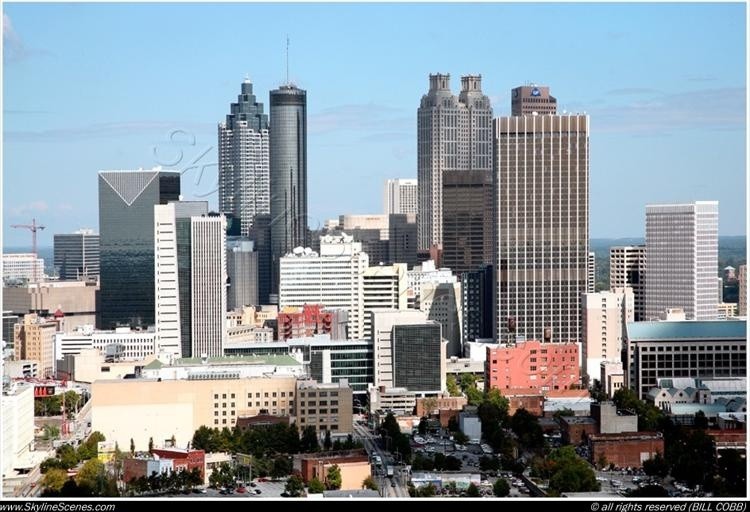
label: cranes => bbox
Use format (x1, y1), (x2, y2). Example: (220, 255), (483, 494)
(9, 218), (47, 282)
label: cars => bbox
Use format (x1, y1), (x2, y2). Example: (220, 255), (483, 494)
(60, 423), (91, 446)
(413, 435), (494, 470)
(602, 464), (693, 498)
(368, 448), (397, 488)
(151, 482), (262, 498)
(474, 472), (530, 496)
